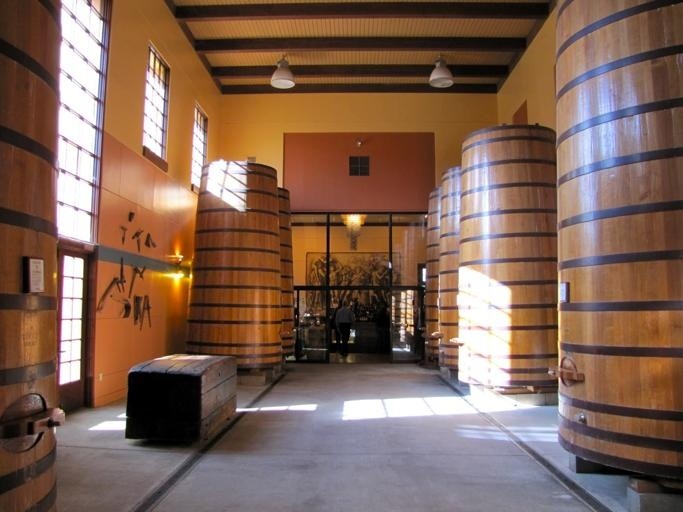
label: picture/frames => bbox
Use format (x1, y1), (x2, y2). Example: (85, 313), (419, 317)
(305, 251), (402, 321)
(29, 255), (45, 294)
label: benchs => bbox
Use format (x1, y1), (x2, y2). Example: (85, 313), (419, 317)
(124, 353), (238, 449)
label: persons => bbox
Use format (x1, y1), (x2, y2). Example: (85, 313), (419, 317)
(308, 253), (391, 306)
(330, 299), (358, 358)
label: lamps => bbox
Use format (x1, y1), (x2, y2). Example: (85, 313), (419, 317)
(270, 55), (297, 91)
(428, 56), (455, 89)
